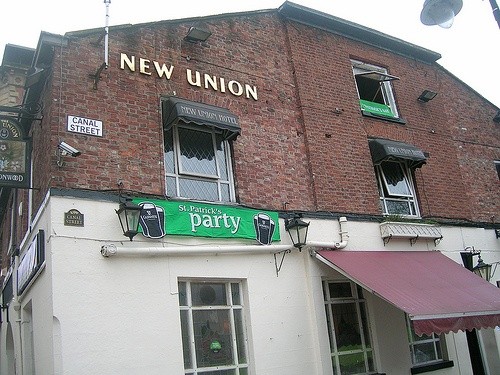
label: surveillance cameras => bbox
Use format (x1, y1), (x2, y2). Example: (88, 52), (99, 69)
(56, 142), (81, 167)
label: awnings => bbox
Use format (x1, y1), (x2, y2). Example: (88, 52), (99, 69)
(309, 249), (500, 338)
(162, 96), (241, 141)
(368, 138), (428, 172)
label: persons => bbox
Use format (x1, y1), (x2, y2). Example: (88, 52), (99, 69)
(199, 285), (238, 337)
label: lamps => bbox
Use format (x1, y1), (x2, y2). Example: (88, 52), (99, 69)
(185, 26), (213, 42)
(114, 182), (144, 242)
(283, 213), (310, 252)
(464, 252), (493, 282)
(418, 90), (438, 102)
(24, 69), (46, 89)
